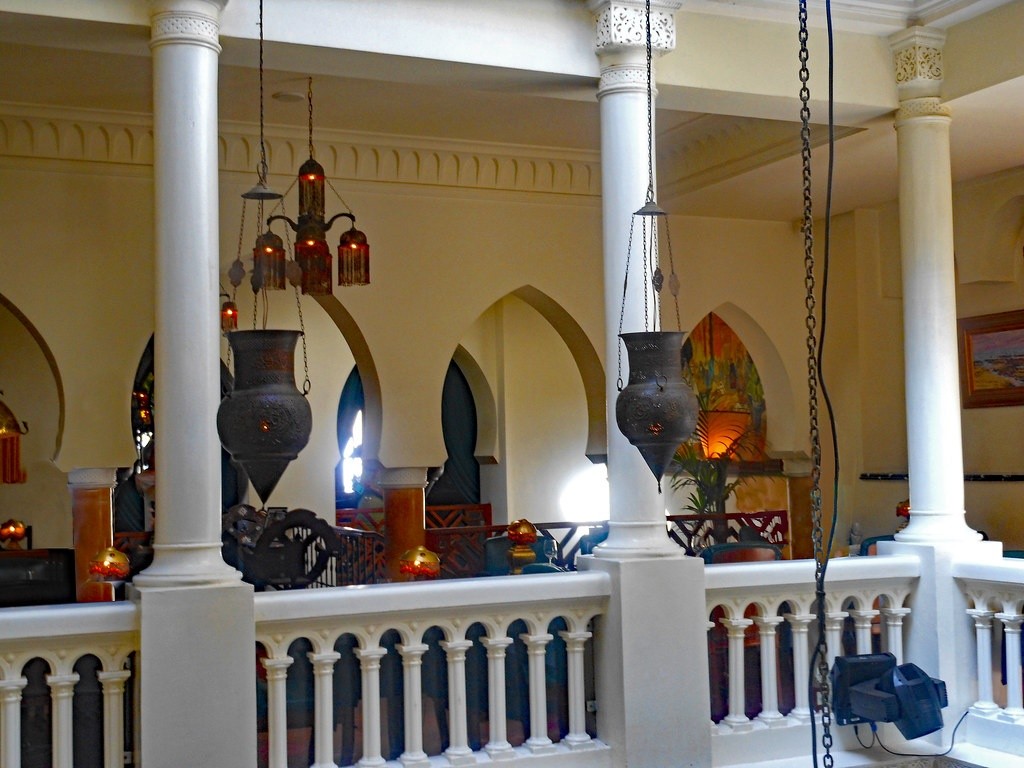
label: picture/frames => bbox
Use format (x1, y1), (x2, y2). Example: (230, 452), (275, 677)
(957, 308), (1024, 409)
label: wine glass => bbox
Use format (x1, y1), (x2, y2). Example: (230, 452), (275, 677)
(544, 538), (558, 564)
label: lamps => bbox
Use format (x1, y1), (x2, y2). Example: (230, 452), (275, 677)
(0, 518), (32, 550)
(250, 77), (370, 297)
(88, 547), (130, 581)
(399, 546), (440, 580)
(0, 390), (30, 485)
(506, 519), (537, 575)
(827, 652), (947, 740)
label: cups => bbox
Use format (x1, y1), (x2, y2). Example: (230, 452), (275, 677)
(692, 536), (709, 553)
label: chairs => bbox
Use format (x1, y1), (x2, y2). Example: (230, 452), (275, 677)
(858, 534), (897, 632)
(694, 544), (784, 717)
